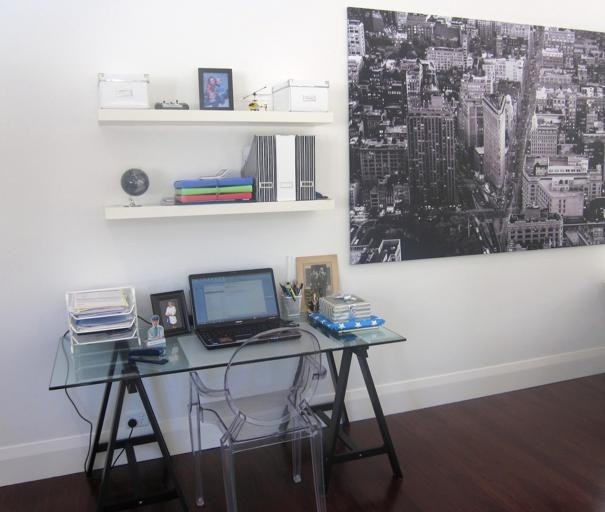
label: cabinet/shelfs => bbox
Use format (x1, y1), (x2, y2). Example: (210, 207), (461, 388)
(65, 285), (141, 353)
(94, 107), (337, 219)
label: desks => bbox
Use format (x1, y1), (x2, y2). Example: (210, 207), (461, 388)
(47, 318), (404, 511)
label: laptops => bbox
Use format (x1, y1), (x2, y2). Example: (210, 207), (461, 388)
(188, 268), (302, 350)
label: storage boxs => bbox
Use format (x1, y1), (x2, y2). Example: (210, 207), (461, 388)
(95, 72), (150, 109)
(270, 78), (330, 112)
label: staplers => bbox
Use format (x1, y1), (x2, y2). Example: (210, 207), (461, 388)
(127, 347), (169, 365)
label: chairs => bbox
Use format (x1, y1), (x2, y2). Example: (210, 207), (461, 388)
(186, 326), (328, 511)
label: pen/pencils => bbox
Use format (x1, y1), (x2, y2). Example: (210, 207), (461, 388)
(279, 279), (303, 301)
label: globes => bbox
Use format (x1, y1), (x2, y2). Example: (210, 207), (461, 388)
(120, 168), (149, 207)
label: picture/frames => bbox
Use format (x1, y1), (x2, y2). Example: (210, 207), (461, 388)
(197, 68), (234, 110)
(295, 254), (341, 314)
(150, 289), (192, 338)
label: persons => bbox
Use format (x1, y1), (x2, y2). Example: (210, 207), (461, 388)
(206, 76), (225, 108)
